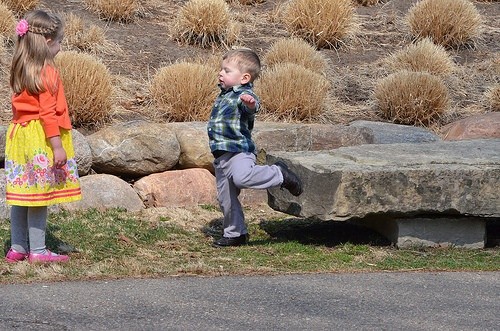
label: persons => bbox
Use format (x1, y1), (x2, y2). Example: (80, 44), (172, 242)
(207, 49), (303, 248)
(4, 8), (82, 265)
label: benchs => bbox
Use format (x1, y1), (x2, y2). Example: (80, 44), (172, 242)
(266, 140), (500, 249)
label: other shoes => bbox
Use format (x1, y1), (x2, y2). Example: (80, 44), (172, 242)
(29, 249), (69, 263)
(4, 248), (29, 263)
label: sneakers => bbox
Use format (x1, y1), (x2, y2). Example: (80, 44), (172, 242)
(212, 233), (250, 248)
(275, 161), (303, 197)
